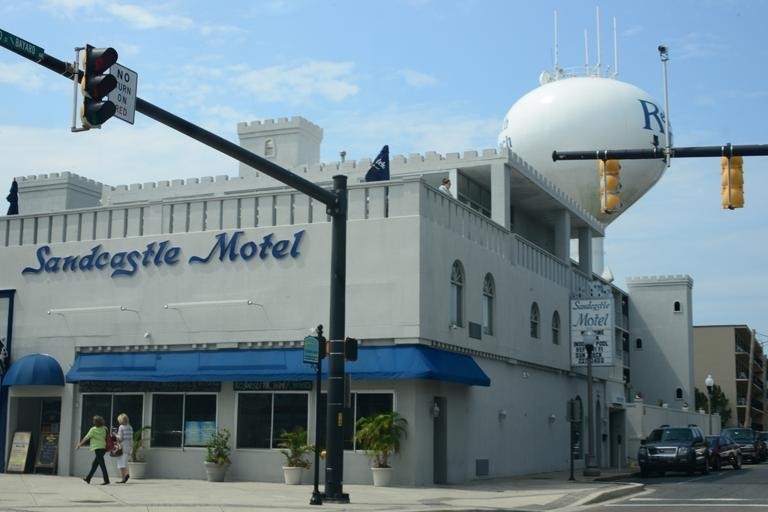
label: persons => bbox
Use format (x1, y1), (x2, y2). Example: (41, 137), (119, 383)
(439, 178), (452, 196)
(112, 412), (133, 482)
(75, 415), (110, 485)
(634, 391), (644, 403)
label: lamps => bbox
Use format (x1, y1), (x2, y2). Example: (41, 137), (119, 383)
(547, 413), (556, 425)
(430, 400), (439, 419)
(497, 408), (506, 424)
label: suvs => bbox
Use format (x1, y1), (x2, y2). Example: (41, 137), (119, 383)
(638, 423), (709, 479)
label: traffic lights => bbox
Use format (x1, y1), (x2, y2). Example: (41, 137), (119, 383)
(79, 42), (119, 131)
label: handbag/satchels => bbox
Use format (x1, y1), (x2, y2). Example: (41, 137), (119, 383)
(109, 444), (123, 456)
(106, 434), (114, 451)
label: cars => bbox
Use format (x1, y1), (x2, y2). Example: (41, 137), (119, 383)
(719, 428), (767, 465)
(704, 435), (742, 473)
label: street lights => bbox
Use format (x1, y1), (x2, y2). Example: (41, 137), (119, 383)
(580, 326), (600, 477)
(704, 375), (713, 435)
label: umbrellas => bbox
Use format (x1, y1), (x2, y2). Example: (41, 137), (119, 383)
(5, 177), (18, 215)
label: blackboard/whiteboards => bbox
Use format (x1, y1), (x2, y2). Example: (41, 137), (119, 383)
(35, 432), (59, 468)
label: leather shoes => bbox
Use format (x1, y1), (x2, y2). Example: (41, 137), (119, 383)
(83, 477), (90, 483)
(125, 474), (129, 482)
(100, 481), (109, 485)
(116, 481), (125, 483)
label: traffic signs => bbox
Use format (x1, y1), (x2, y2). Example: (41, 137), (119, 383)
(0, 29), (45, 64)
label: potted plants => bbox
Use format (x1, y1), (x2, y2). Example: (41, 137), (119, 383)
(347, 411), (409, 488)
(200, 423), (236, 483)
(276, 424), (313, 485)
(126, 424), (154, 479)
